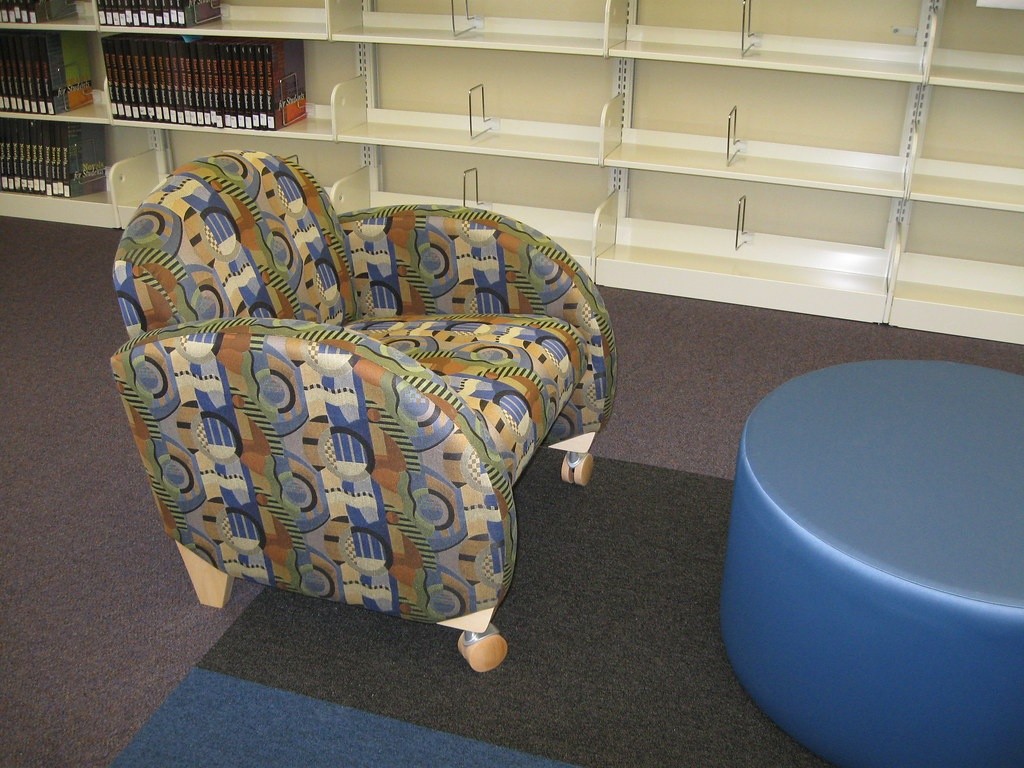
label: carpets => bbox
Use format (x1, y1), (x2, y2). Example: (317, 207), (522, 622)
(110, 446), (843, 768)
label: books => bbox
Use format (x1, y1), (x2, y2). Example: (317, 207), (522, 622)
(101, 33), (308, 132)
(0, 0), (79, 24)
(0, 29), (95, 115)
(0, 118), (108, 198)
(96, 0), (222, 29)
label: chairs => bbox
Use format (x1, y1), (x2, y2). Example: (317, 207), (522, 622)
(113, 150), (618, 670)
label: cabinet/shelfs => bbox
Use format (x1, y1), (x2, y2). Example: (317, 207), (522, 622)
(0, 0), (1024, 344)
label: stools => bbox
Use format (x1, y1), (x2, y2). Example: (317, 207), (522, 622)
(722, 359), (1024, 768)
